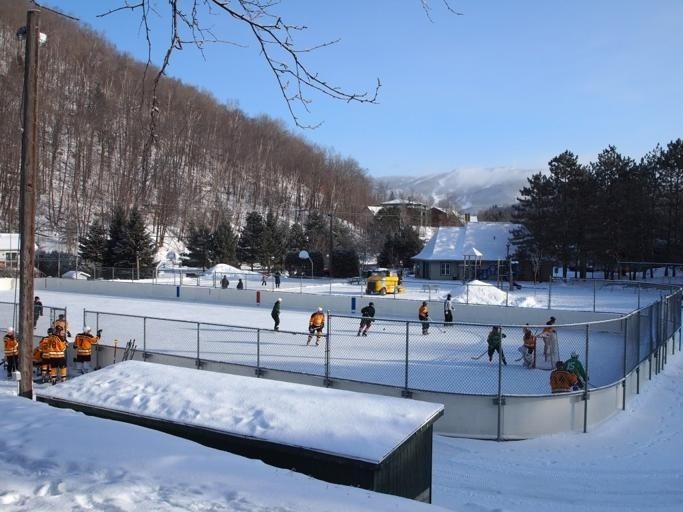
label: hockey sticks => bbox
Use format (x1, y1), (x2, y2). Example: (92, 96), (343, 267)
(515, 328), (538, 362)
(471, 350), (488, 362)
(427, 315), (446, 335)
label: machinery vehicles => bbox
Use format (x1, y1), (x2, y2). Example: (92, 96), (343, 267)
(365, 268), (406, 296)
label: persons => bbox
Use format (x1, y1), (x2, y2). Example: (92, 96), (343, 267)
(274, 270), (281, 289)
(518, 316), (557, 368)
(357, 302), (375, 336)
(237, 279), (243, 289)
(564, 352), (589, 391)
(271, 297), (282, 331)
(443, 294), (454, 326)
(305, 307), (326, 346)
(220, 275), (230, 288)
(33, 297), (43, 328)
(487, 325), (508, 366)
(419, 301), (430, 335)
(550, 361), (577, 393)
(3, 315), (102, 385)
(262, 274), (268, 286)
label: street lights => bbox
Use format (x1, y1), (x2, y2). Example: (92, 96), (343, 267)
(13, 10), (46, 403)
(326, 213), (335, 278)
(504, 240), (511, 264)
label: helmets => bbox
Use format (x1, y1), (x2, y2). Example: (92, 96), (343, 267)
(571, 352), (578, 358)
(83, 326), (91, 333)
(48, 325), (63, 336)
(7, 327), (14, 335)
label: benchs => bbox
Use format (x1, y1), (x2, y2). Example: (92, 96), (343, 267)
(15, 358), (51, 377)
